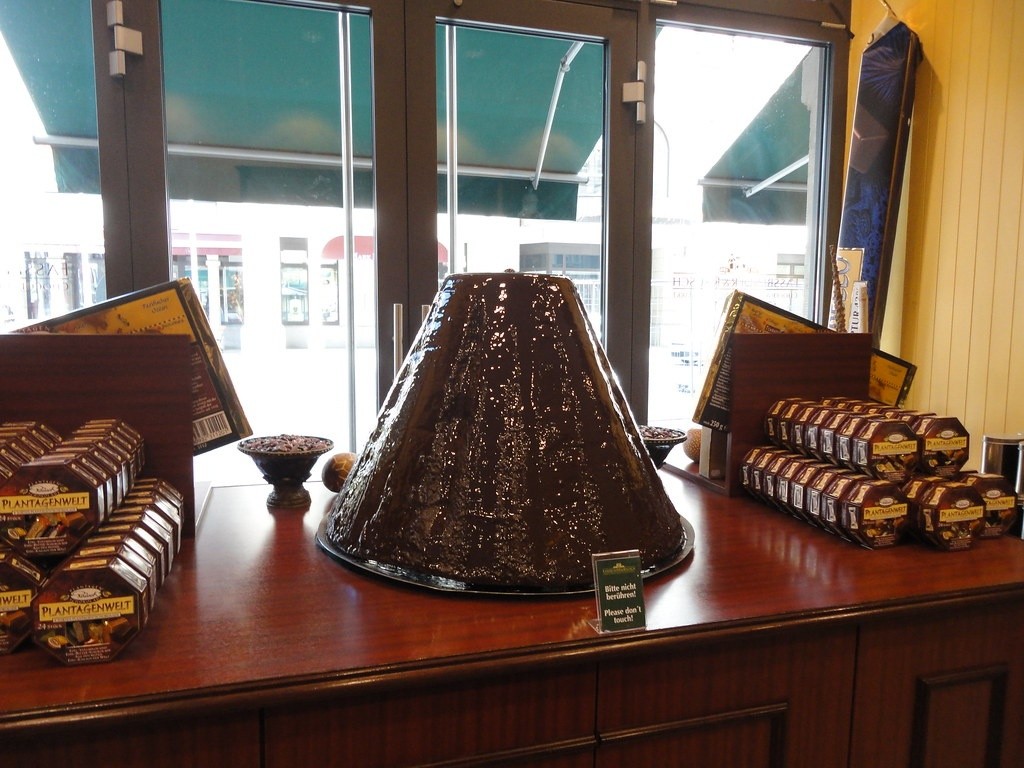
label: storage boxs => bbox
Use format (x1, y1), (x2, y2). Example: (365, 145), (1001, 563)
(1, 277), (253, 665)
(691, 289), (1018, 553)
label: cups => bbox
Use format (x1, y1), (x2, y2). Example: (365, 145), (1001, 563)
(982, 433), (1024, 495)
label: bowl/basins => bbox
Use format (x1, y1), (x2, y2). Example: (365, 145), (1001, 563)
(636, 426), (687, 467)
(236, 435), (334, 509)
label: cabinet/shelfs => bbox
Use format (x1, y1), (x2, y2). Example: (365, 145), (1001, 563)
(1, 446), (1024, 768)
